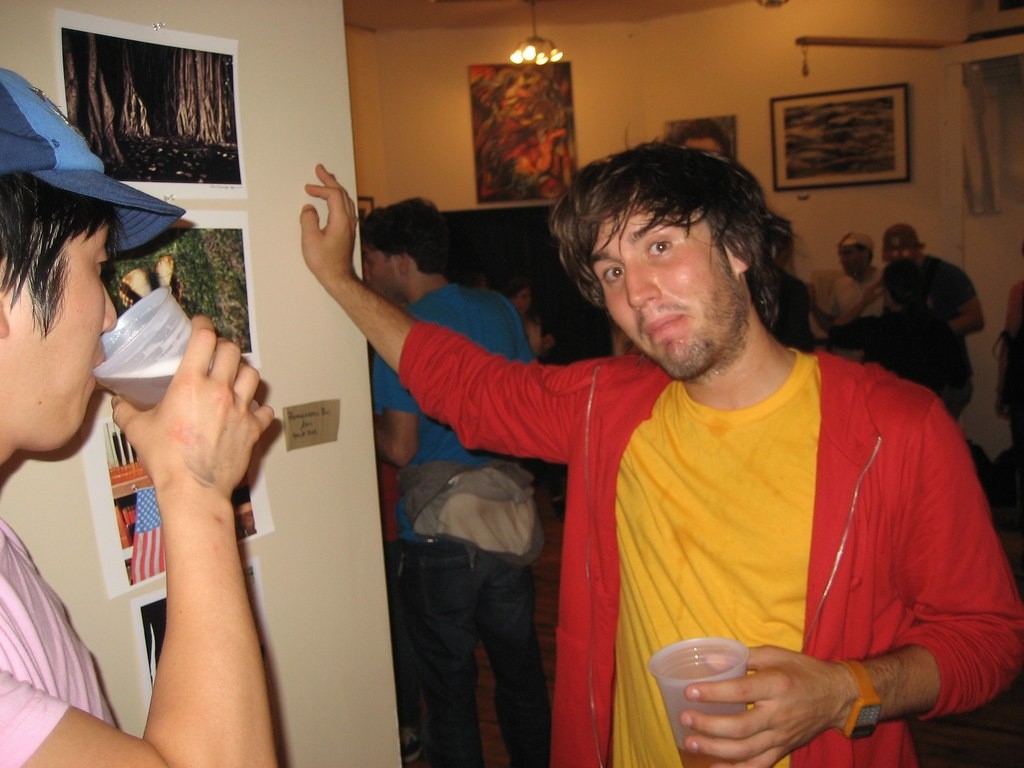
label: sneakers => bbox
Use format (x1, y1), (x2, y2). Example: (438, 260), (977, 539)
(399, 727), (424, 761)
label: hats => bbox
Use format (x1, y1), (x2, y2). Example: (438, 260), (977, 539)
(0, 68), (187, 252)
(838, 231), (874, 257)
(881, 223), (925, 249)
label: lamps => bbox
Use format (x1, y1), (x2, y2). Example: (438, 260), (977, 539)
(509, 1), (564, 65)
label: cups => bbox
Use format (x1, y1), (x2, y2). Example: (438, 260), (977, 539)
(648, 637), (750, 768)
(92, 285), (260, 414)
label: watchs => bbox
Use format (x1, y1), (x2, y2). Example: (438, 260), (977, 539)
(832, 658), (882, 740)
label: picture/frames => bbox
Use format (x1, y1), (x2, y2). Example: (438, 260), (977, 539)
(769, 82), (909, 191)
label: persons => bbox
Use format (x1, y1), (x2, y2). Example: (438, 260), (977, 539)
(301, 141), (1024, 768)
(0, 69), (277, 768)
